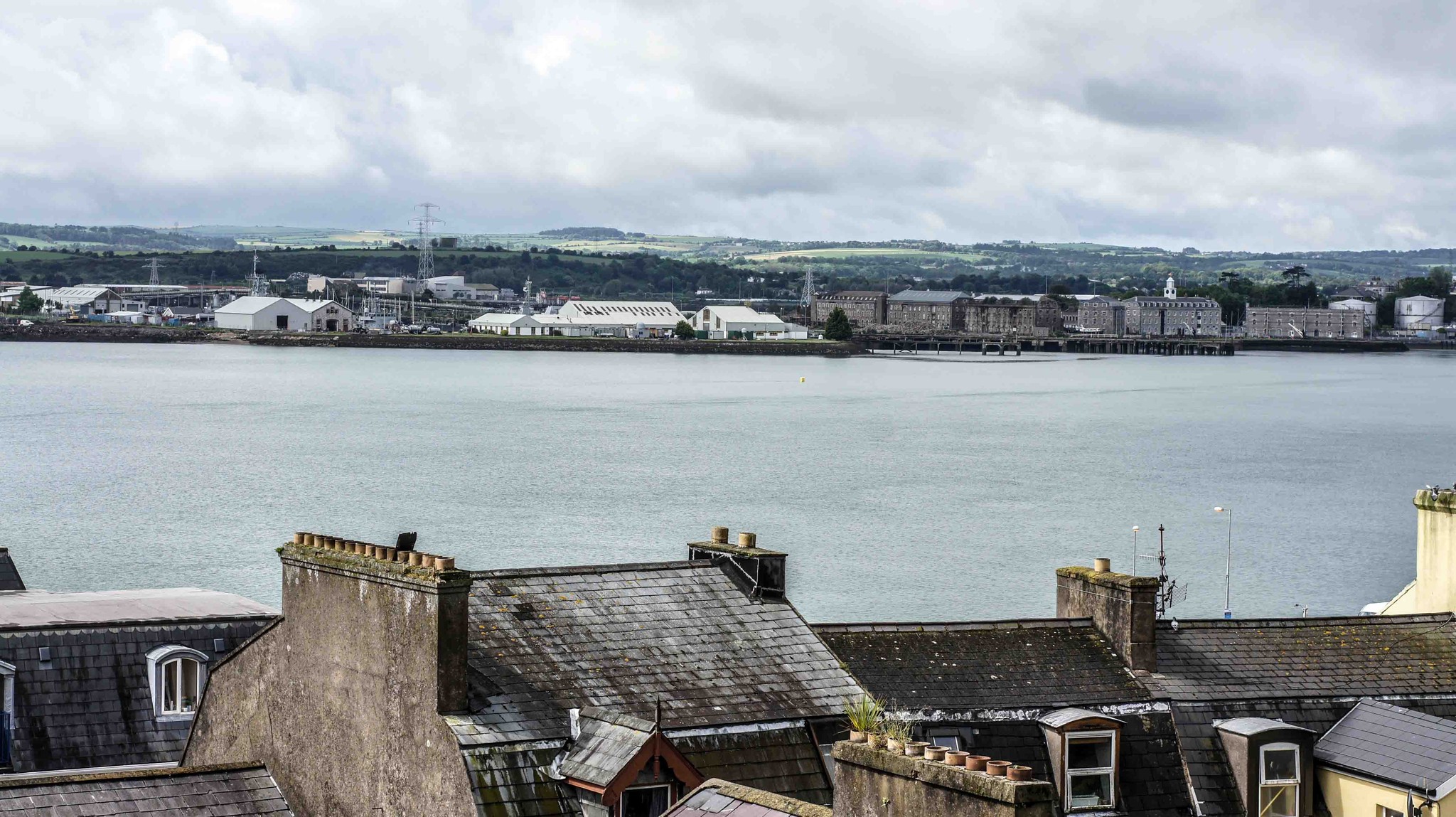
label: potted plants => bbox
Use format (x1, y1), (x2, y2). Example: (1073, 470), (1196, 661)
(838, 683), (889, 742)
(863, 717), (888, 748)
(884, 709), (922, 753)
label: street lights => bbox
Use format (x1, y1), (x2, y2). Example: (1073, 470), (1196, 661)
(1295, 604), (1308, 618)
(1214, 507), (1232, 618)
(803, 304), (809, 326)
(1132, 526), (1140, 576)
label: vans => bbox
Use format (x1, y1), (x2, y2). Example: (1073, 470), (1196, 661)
(19, 320), (35, 326)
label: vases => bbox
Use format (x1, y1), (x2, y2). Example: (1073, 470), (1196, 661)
(905, 738), (1034, 782)
